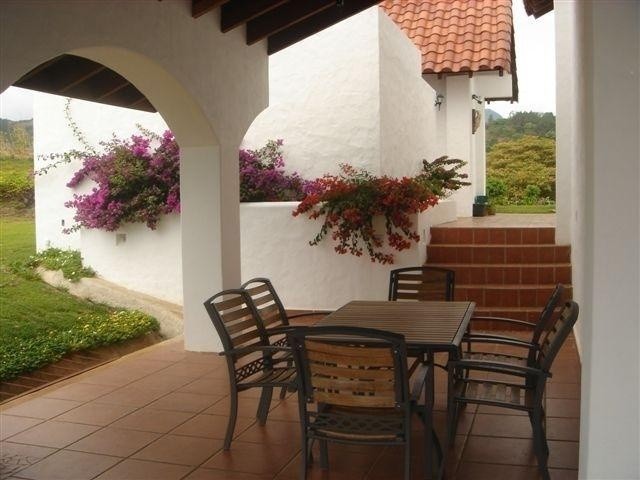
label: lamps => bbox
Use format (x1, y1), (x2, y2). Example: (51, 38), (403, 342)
(434, 93), (444, 111)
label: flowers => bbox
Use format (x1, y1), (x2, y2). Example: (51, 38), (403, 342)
(28, 88), (297, 236)
(288, 154), (475, 263)
